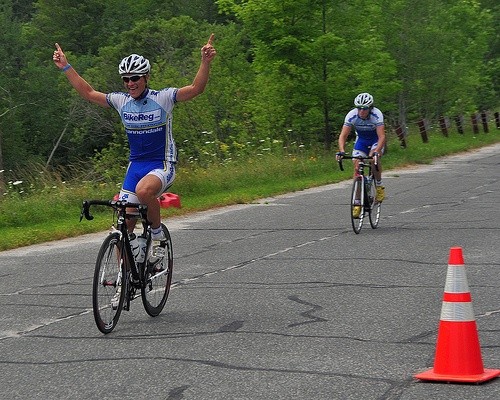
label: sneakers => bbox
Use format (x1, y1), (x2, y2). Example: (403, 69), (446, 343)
(146, 235), (168, 267)
(352, 203), (362, 217)
(375, 186), (385, 203)
(111, 286), (127, 308)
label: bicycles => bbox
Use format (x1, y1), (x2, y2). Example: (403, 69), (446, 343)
(334, 151), (381, 235)
(78, 199), (174, 334)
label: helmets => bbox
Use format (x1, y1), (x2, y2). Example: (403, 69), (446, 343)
(354, 93), (374, 109)
(118, 54), (151, 75)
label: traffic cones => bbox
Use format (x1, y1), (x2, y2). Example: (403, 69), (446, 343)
(415, 247), (499, 385)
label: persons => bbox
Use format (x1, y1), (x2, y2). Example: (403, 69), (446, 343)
(53, 34), (216, 307)
(336, 93), (386, 217)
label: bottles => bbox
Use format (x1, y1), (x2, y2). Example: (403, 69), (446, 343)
(135, 234), (147, 262)
(367, 174), (373, 187)
(128, 233), (141, 261)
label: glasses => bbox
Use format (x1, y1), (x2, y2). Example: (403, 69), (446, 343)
(358, 107), (369, 110)
(120, 74), (148, 82)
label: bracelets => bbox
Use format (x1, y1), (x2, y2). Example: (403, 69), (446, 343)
(63, 64), (71, 71)
